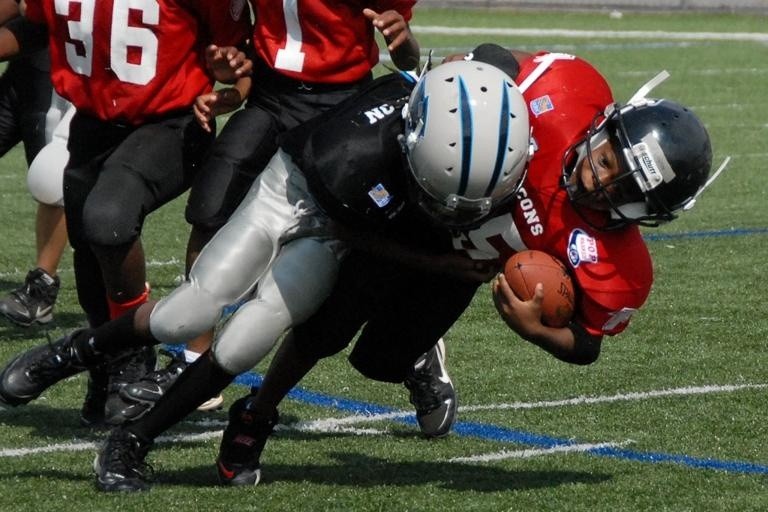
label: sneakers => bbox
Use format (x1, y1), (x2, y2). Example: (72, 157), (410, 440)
(0, 267), (60, 326)
(2, 342), (230, 492)
(215, 394), (280, 487)
(404, 337), (457, 438)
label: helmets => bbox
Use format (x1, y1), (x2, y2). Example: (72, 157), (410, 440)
(400, 60), (531, 230)
(608, 99), (713, 215)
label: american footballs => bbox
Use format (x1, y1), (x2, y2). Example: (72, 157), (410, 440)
(504, 248), (576, 327)
